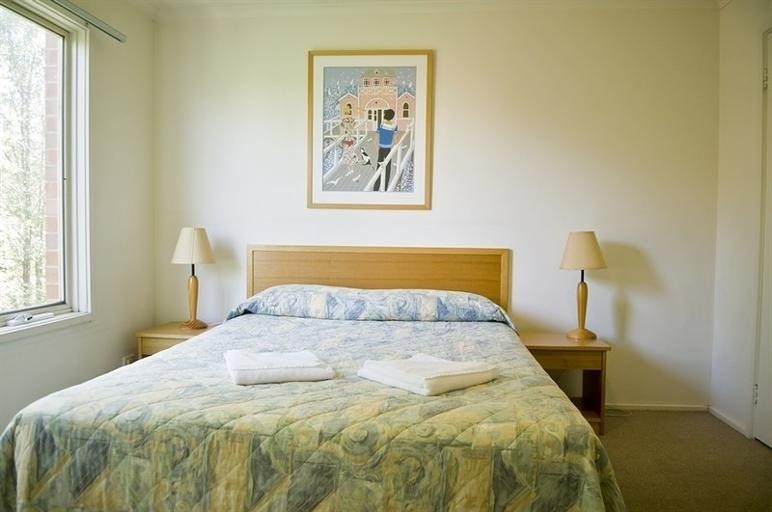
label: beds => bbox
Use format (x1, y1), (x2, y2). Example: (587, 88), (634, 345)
(0, 242), (622, 511)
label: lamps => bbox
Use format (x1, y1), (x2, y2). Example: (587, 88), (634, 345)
(174, 225), (212, 329)
(560, 229), (610, 343)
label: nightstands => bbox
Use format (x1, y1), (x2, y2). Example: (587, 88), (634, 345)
(136, 320), (212, 362)
(521, 332), (614, 437)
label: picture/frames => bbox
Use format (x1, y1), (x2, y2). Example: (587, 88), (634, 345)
(306, 48), (434, 211)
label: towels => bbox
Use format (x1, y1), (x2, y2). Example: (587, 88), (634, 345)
(225, 346), (334, 386)
(361, 353), (498, 396)
